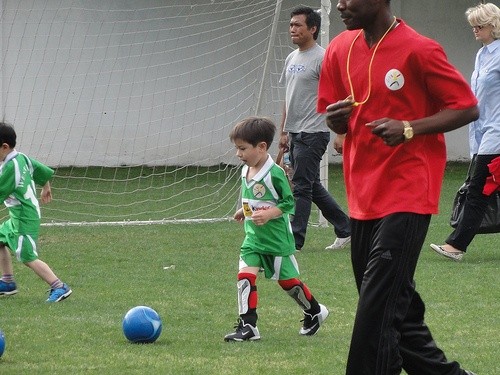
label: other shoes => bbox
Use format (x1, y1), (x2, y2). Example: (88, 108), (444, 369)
(429, 242), (463, 261)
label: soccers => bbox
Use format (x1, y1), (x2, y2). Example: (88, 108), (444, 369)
(122, 305), (162, 344)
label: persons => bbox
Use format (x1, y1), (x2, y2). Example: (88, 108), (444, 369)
(224, 118), (330, 342)
(430, 4), (500, 260)
(0, 122), (73, 305)
(277, 5), (352, 253)
(315, 0), (473, 375)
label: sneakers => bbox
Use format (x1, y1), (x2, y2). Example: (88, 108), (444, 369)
(325, 235), (352, 251)
(44, 282), (72, 303)
(297, 303), (329, 337)
(222, 318), (261, 343)
(0, 277), (18, 297)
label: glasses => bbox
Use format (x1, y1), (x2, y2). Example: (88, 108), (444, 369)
(471, 25), (484, 31)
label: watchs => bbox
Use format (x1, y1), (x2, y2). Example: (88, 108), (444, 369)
(402, 121), (414, 140)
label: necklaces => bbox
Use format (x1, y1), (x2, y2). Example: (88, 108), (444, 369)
(346, 15), (396, 108)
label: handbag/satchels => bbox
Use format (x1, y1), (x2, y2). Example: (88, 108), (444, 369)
(448, 152), (500, 234)
